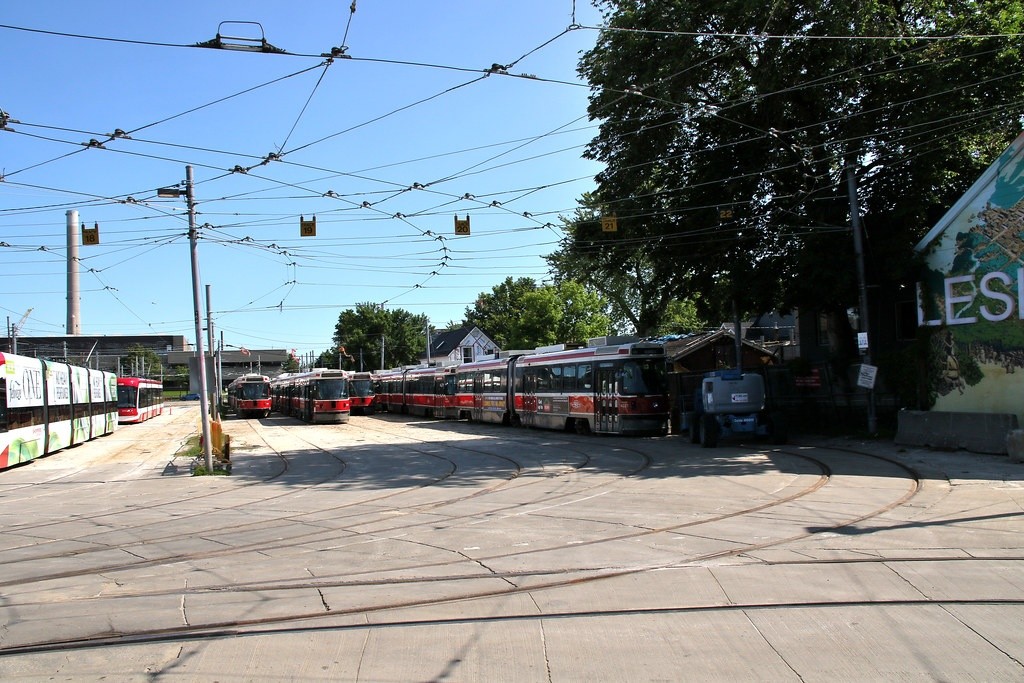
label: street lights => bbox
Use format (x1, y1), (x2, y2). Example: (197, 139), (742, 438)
(214, 344), (251, 420)
(158, 188), (214, 474)
(187, 343), (209, 347)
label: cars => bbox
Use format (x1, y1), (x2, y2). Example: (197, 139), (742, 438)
(180, 393), (200, 401)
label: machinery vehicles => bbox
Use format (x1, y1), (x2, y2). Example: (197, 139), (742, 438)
(667, 366), (787, 448)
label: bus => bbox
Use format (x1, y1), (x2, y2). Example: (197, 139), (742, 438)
(116, 377), (164, 422)
(0, 351), (118, 468)
(227, 335), (673, 435)
